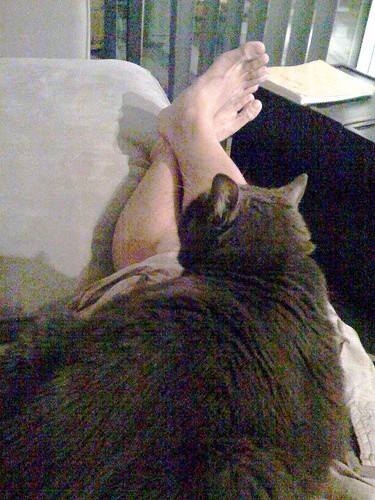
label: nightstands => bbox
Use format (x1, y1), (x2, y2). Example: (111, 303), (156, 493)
(229, 61), (375, 343)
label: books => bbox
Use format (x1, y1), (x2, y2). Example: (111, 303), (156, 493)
(258, 59), (371, 107)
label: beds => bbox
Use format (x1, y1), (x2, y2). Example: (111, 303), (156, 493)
(1, 56), (342, 325)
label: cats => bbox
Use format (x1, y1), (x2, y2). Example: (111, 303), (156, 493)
(0, 171), (350, 500)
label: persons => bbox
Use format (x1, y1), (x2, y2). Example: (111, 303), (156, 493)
(85, 41), (375, 500)
